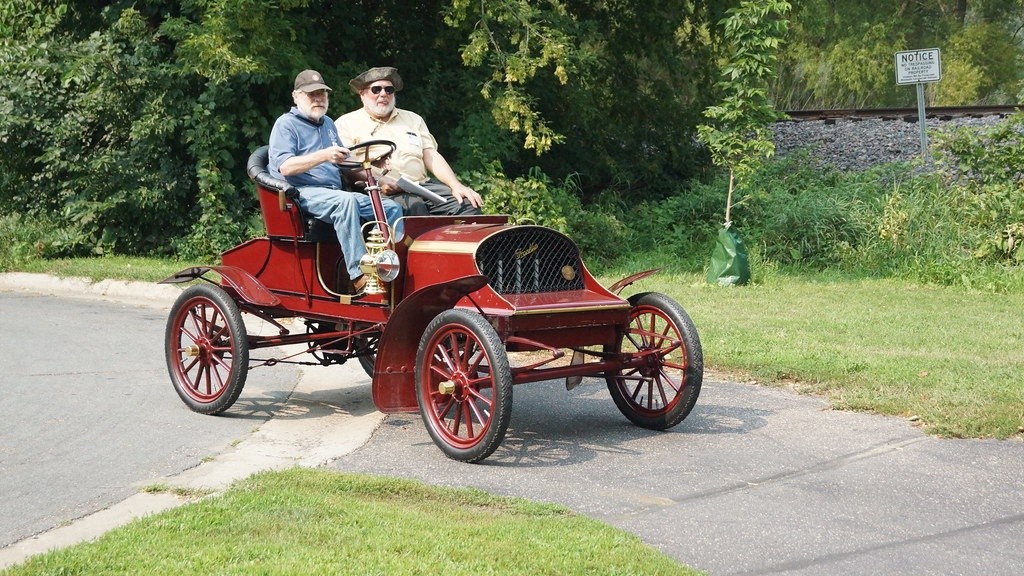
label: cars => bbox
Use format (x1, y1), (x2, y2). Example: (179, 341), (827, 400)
(157, 138), (704, 464)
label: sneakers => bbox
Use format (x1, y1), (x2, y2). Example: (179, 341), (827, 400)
(354, 274), (388, 297)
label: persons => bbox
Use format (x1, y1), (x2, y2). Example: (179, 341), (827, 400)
(333, 66), (484, 216)
(268, 69), (405, 293)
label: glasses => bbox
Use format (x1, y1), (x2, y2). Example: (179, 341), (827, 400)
(362, 86), (397, 95)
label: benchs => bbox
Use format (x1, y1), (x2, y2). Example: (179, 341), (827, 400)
(247, 145), (345, 243)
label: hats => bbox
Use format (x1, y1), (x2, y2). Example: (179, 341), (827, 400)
(295, 69), (332, 92)
(348, 67), (404, 94)
